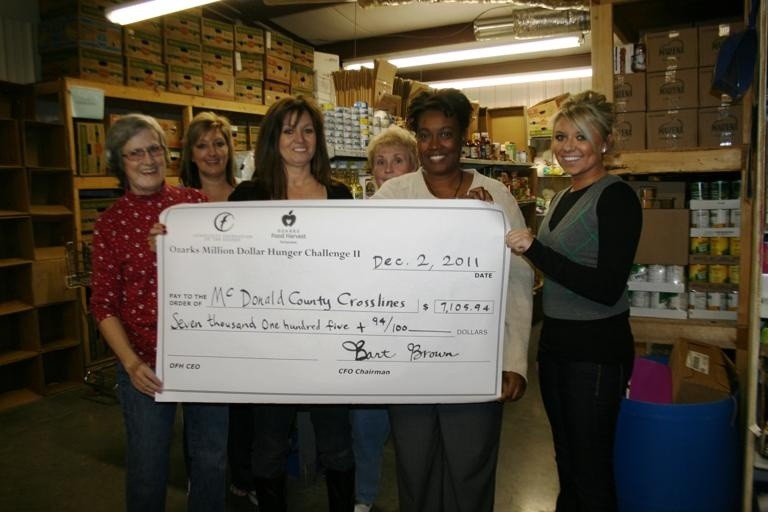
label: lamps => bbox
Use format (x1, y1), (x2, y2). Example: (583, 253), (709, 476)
(342, 33), (585, 72)
(429, 69), (592, 90)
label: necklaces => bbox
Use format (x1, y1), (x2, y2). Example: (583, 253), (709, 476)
(425, 171), (463, 198)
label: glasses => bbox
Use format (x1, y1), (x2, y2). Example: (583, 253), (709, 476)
(117, 145), (166, 162)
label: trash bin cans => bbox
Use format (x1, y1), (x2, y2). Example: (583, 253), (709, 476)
(614, 357), (741, 512)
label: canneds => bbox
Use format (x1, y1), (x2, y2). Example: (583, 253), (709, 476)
(690, 264), (707, 282)
(669, 292), (689, 309)
(708, 290), (726, 310)
(730, 209), (740, 228)
(727, 290), (740, 311)
(647, 264), (665, 282)
(710, 209), (730, 227)
(689, 182), (711, 200)
(651, 292), (669, 310)
(728, 265), (740, 283)
(658, 198), (675, 209)
(729, 237), (741, 256)
(730, 180), (742, 199)
(641, 199), (658, 210)
(710, 237), (728, 255)
(638, 186), (658, 199)
(665, 265), (688, 282)
(689, 290), (707, 309)
(711, 180), (730, 200)
(690, 209), (711, 228)
(708, 265), (728, 283)
(691, 237), (710, 254)
(632, 291), (651, 307)
(631, 265), (649, 281)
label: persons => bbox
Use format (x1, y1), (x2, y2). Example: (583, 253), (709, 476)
(178, 111), (256, 499)
(147, 97), (355, 512)
(505, 91), (642, 512)
(368, 89), (535, 512)
(351, 124), (421, 512)
(90, 112), (230, 512)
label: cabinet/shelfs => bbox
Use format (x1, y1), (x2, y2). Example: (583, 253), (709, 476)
(589, 0), (768, 512)
(330, 148), (544, 294)
(0, 81), (83, 411)
(32, 78), (270, 386)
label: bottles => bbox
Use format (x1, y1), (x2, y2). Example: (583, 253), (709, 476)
(460, 131), (528, 163)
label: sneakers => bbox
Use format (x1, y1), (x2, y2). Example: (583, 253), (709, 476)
(229, 481), (258, 506)
